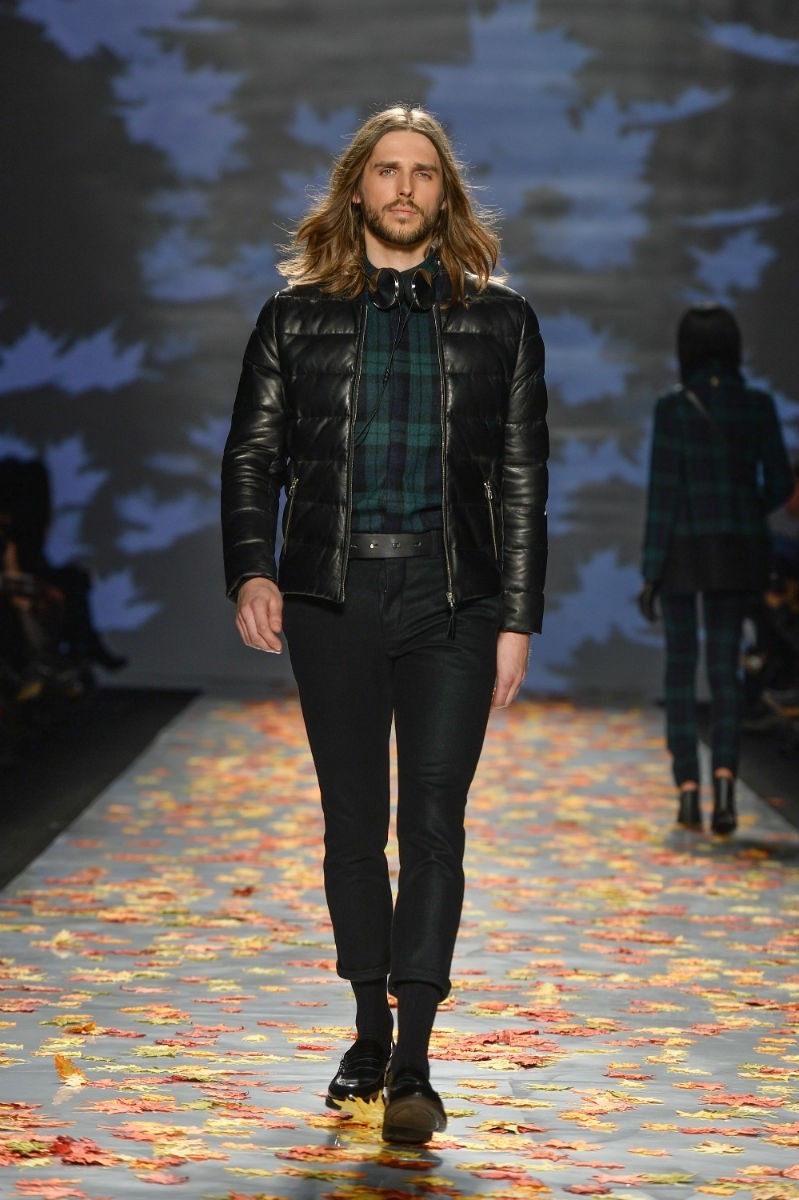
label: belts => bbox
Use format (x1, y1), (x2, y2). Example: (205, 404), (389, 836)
(347, 530), (445, 559)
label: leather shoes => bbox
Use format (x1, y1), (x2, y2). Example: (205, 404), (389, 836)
(381, 1070), (447, 1145)
(328, 1038), (396, 1102)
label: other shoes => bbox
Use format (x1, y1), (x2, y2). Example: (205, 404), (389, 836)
(711, 776), (736, 833)
(676, 790), (698, 827)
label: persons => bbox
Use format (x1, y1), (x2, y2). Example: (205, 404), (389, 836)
(737, 560), (799, 719)
(637, 303), (795, 832)
(0, 556), (117, 760)
(220, 105), (552, 1145)
